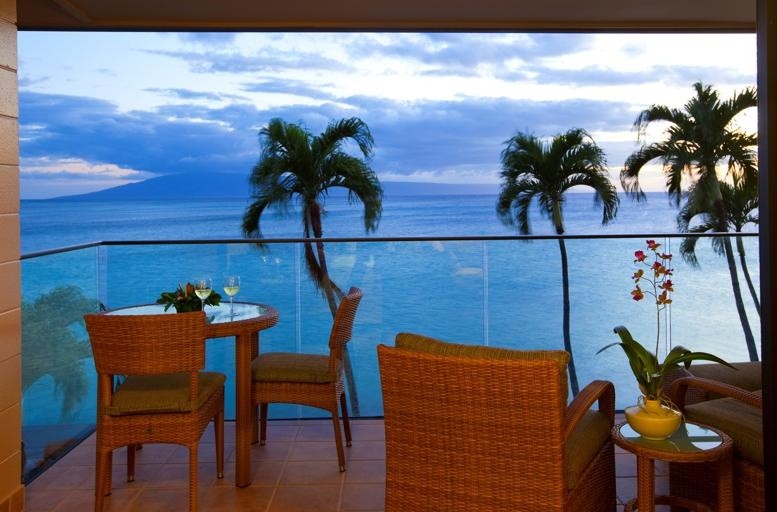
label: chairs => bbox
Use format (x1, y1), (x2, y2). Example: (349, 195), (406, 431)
(666, 375), (764, 512)
(375, 329), (616, 512)
(83, 309), (228, 511)
(247, 285), (363, 474)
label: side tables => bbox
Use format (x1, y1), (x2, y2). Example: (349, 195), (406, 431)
(611, 421), (736, 512)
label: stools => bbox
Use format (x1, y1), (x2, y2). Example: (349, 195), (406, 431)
(659, 359), (762, 405)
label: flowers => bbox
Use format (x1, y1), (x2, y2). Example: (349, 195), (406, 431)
(158, 283), (222, 313)
(596, 239), (741, 401)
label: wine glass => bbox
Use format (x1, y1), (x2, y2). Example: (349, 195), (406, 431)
(194, 276), (240, 315)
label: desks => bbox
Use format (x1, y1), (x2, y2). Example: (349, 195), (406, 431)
(95, 299), (279, 499)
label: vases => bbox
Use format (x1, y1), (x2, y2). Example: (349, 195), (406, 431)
(624, 395), (682, 442)
